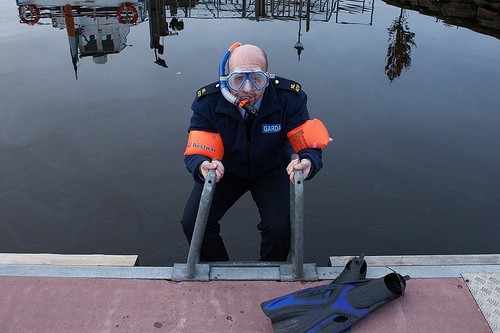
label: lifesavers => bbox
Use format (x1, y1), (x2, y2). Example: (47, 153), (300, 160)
(183, 130), (224, 161)
(19, 4), (40, 25)
(287, 118), (333, 154)
(116, 5), (138, 24)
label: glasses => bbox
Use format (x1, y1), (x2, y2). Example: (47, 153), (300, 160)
(226, 70), (270, 93)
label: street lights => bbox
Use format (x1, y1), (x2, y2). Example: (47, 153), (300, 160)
(293, 0), (305, 63)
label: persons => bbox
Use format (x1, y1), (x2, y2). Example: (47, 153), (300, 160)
(181, 43), (333, 262)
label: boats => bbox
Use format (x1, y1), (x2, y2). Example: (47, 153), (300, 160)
(15, 1), (149, 80)
